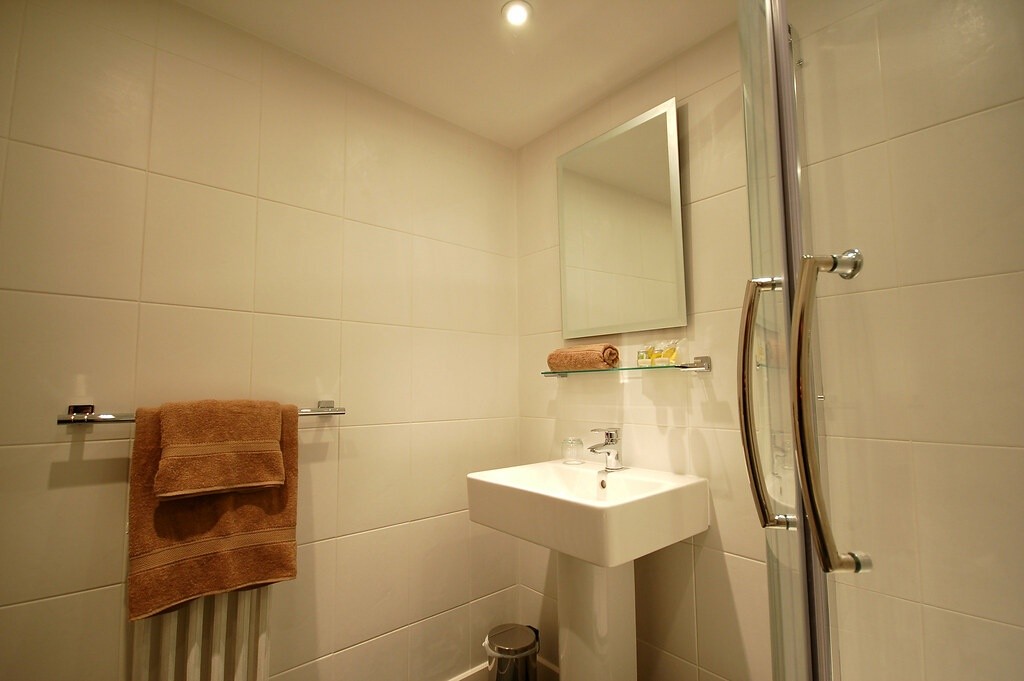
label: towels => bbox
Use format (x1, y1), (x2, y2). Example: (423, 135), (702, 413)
(122, 400), (297, 622)
(547, 344), (619, 371)
(152, 400), (287, 498)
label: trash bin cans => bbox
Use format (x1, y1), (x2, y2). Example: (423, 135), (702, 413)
(488, 623), (538, 681)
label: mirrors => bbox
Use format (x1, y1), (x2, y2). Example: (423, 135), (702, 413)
(555, 96), (687, 340)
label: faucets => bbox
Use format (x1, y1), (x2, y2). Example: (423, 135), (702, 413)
(588, 427), (623, 472)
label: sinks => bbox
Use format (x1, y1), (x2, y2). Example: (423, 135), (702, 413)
(465, 457), (710, 566)
